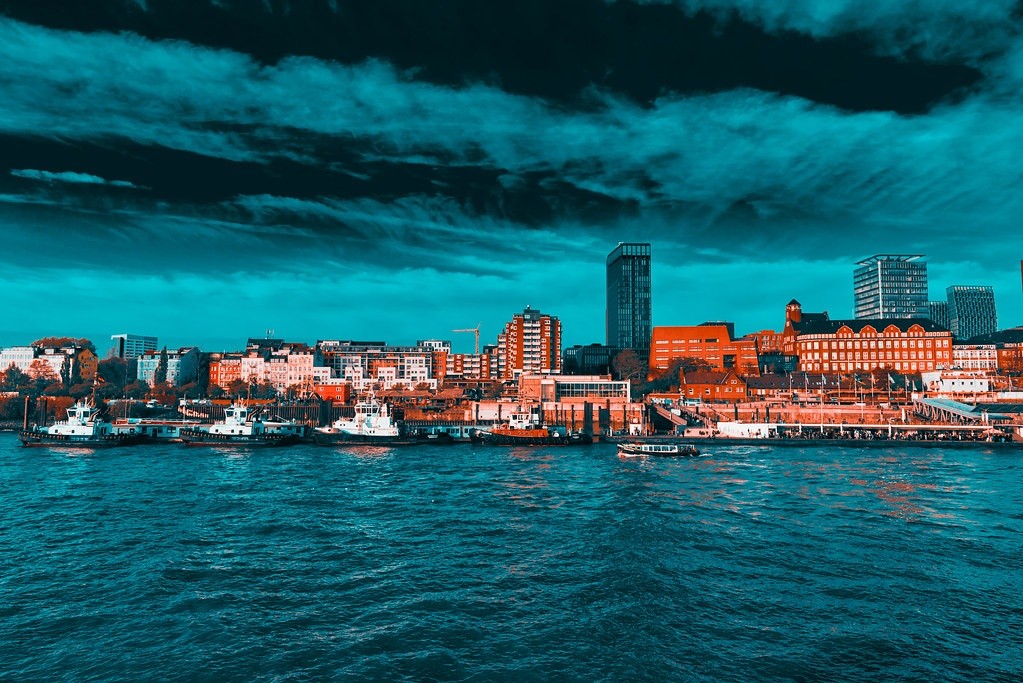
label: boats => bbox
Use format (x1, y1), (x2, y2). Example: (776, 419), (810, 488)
(313, 373), (453, 446)
(468, 398), (592, 446)
(19, 387), (149, 447)
(179, 395), (298, 446)
(600, 426), (630, 443)
(616, 436), (700, 458)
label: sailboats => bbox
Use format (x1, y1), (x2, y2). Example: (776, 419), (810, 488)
(111, 375), (319, 442)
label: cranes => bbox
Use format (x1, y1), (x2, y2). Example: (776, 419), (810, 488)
(452, 322), (481, 354)
